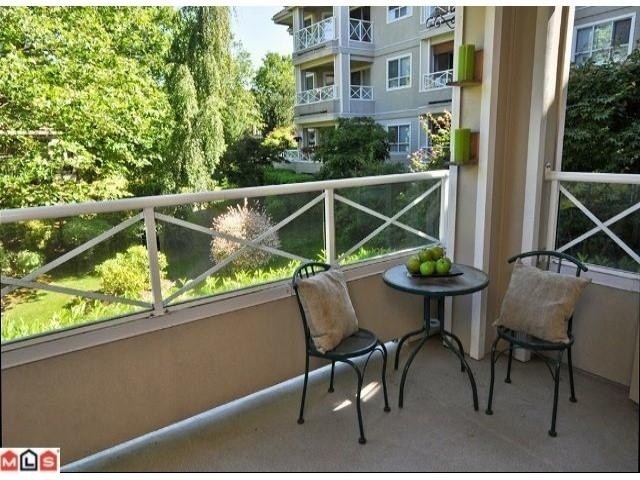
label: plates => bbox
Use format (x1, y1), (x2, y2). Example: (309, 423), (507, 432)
(404, 263), (464, 279)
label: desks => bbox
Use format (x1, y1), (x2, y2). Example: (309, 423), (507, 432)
(382, 262), (491, 412)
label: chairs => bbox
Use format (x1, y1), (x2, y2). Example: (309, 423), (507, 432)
(293, 262), (392, 444)
(486, 250), (588, 438)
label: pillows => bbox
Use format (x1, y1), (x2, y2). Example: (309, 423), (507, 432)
(296, 263), (359, 355)
(492, 258), (590, 345)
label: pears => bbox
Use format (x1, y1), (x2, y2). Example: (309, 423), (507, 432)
(405, 255), (420, 273)
(436, 259), (451, 274)
(420, 261), (434, 276)
(431, 246), (444, 260)
(419, 249), (432, 262)
